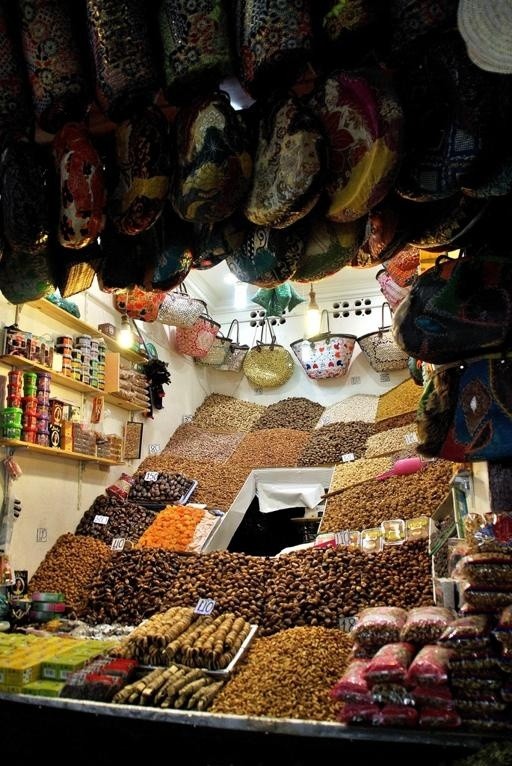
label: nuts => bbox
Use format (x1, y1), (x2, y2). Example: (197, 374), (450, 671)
(26, 376), (512, 731)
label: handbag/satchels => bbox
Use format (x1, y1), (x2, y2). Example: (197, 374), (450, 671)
(113, 283), (408, 386)
(376, 244), (512, 460)
(0, 1), (512, 304)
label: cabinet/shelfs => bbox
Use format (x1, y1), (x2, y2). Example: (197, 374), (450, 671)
(0, 298), (151, 466)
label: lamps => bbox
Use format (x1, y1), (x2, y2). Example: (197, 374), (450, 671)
(306, 283), (321, 337)
